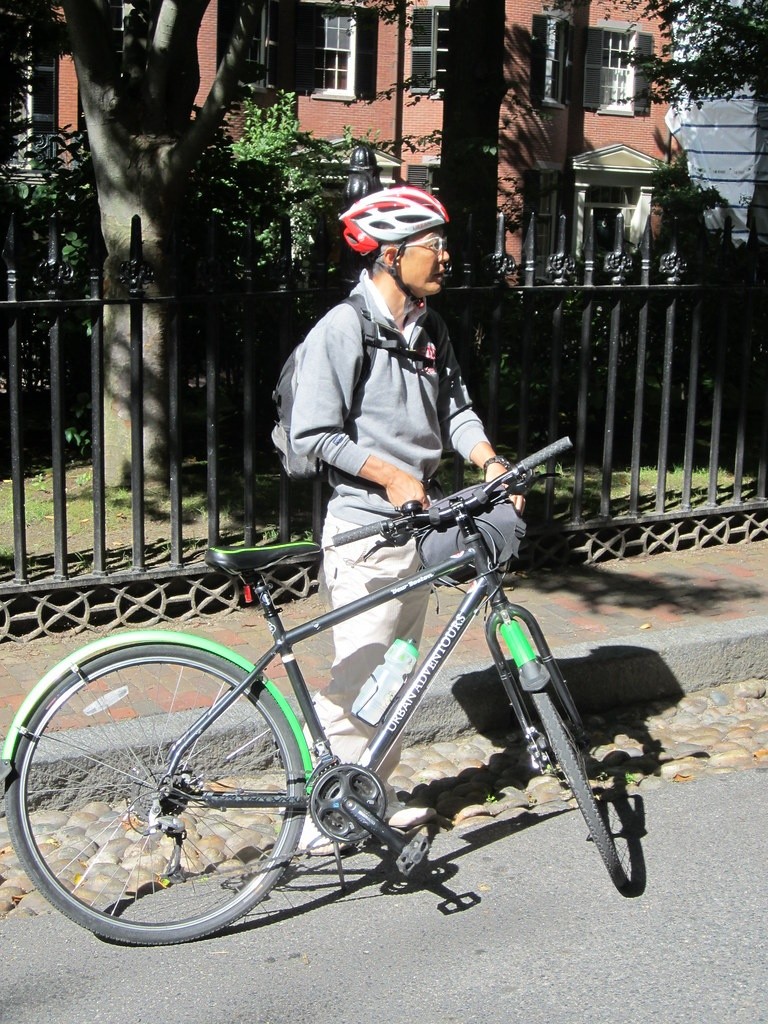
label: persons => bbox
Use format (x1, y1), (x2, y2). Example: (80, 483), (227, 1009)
(292, 187), (523, 856)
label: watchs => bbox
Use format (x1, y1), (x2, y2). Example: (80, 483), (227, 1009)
(484, 456), (512, 476)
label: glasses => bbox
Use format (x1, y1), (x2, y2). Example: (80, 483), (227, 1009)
(397, 236), (448, 256)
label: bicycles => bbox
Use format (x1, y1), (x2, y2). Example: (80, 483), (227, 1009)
(0, 435), (630, 950)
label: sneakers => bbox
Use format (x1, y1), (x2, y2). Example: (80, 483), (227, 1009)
(278, 807), (344, 855)
(377, 786), (436, 828)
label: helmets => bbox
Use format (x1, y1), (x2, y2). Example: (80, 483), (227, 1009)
(337, 185), (450, 255)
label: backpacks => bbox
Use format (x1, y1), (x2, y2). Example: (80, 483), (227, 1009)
(270, 295), (451, 480)
